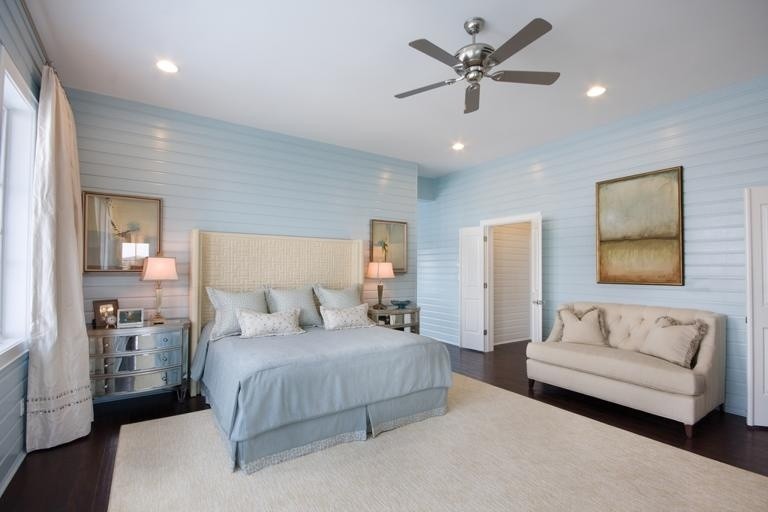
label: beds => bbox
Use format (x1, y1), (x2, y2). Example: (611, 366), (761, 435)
(188, 228), (453, 477)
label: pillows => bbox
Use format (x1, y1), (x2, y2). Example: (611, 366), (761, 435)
(639, 317), (709, 369)
(264, 286), (323, 329)
(556, 306), (610, 347)
(236, 308), (306, 336)
(312, 284), (361, 313)
(204, 287), (268, 341)
(319, 304), (377, 330)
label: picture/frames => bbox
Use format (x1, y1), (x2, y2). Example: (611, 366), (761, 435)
(370, 219), (408, 275)
(93, 299), (119, 327)
(595, 165), (685, 287)
(82, 190), (161, 273)
(117, 309), (143, 328)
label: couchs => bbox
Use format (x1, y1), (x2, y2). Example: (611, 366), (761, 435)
(524, 302), (727, 439)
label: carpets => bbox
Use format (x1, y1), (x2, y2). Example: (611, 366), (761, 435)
(108, 370), (768, 512)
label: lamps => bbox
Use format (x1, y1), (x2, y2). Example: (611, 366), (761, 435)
(365, 263), (394, 309)
(121, 243), (149, 268)
(141, 257), (178, 325)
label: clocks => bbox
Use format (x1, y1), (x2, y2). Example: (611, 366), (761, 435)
(105, 314), (116, 329)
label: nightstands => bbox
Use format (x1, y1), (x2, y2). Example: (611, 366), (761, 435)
(369, 307), (419, 336)
(86, 318), (191, 403)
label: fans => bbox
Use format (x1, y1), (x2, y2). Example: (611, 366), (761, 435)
(393, 17), (561, 115)
